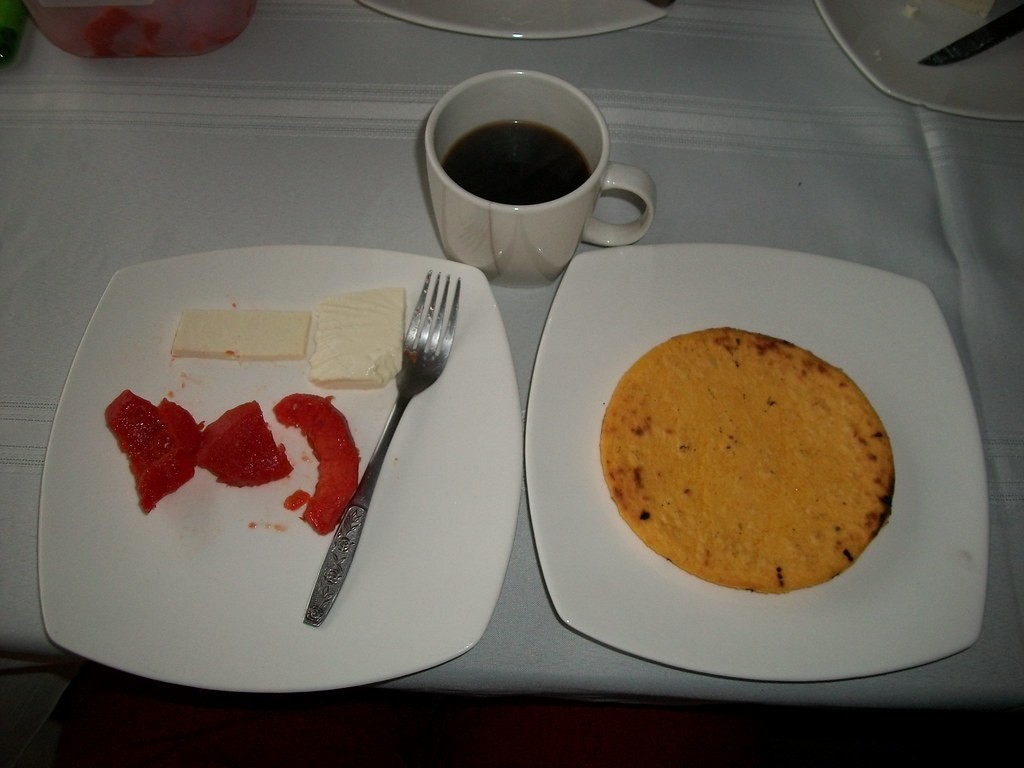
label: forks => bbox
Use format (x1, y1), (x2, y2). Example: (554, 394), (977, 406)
(304, 269), (469, 628)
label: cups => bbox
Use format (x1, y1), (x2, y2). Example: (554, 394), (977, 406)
(425, 67), (656, 287)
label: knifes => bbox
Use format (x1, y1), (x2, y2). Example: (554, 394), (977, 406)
(916, 4), (1024, 71)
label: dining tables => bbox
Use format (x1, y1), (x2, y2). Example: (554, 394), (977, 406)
(0, 0), (1024, 709)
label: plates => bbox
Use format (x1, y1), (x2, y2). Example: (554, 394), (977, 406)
(38, 246), (524, 694)
(812, 1), (1024, 126)
(356, 1), (674, 37)
(525, 240), (989, 683)
